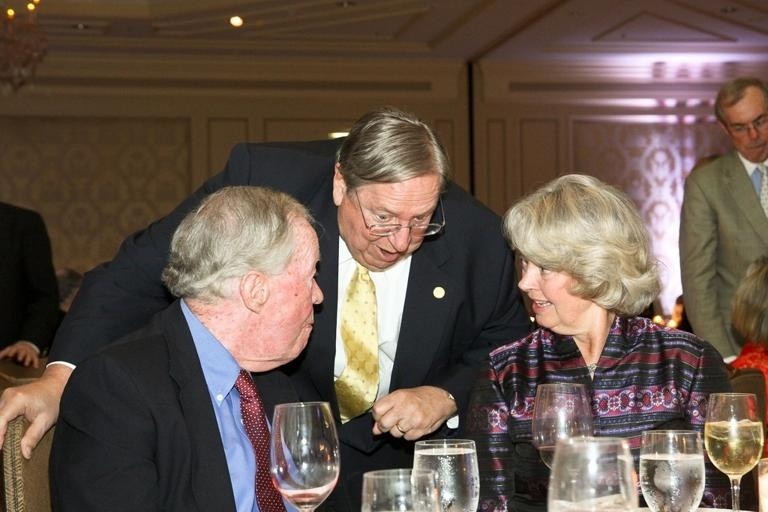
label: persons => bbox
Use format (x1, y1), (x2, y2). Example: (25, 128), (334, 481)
(729, 256), (767, 462)
(465, 173), (755, 512)
(38, 106), (532, 512)
(0, 202), (62, 372)
(679, 77), (768, 362)
(50, 187), (324, 512)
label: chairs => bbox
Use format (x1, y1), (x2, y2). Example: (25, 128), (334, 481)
(3, 410), (55, 512)
(0, 354), (49, 400)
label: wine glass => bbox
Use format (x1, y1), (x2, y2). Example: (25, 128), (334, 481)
(531, 382), (594, 471)
(360, 468), (441, 511)
(412, 439), (481, 511)
(547, 435), (639, 512)
(640, 429), (706, 512)
(705, 392), (764, 511)
(268, 400), (340, 511)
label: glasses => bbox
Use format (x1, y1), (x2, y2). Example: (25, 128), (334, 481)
(354, 187), (447, 239)
(723, 114), (766, 133)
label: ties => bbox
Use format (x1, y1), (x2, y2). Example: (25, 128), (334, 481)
(750, 165), (763, 203)
(335, 265), (379, 424)
(236, 372), (288, 512)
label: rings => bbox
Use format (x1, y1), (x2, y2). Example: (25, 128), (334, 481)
(396, 423), (406, 434)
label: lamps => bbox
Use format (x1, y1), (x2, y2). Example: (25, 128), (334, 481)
(0, 0), (52, 97)
